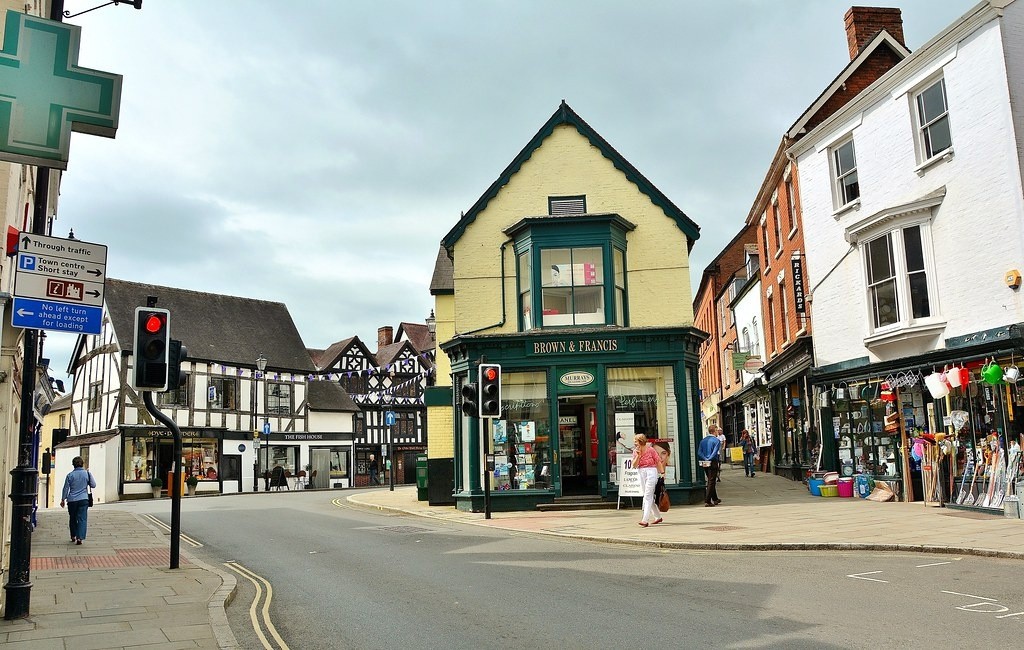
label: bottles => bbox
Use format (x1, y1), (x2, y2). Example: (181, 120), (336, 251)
(857, 438), (863, 446)
(885, 400), (895, 415)
(924, 366), (961, 399)
(861, 406), (867, 418)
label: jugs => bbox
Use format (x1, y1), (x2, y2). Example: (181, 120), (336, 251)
(959, 368), (968, 392)
(984, 361), (1010, 386)
(977, 364), (993, 383)
(1006, 364), (1024, 383)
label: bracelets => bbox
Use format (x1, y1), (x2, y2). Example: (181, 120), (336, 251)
(661, 472), (665, 474)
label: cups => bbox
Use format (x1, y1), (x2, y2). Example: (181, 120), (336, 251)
(881, 437), (890, 445)
(853, 412), (861, 419)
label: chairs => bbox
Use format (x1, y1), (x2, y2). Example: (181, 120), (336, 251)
(264, 470), (317, 492)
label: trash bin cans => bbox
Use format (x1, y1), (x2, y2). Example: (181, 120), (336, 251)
(416, 453), (428, 501)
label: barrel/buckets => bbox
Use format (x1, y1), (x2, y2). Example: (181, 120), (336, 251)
(810, 479), (824, 495)
(819, 384), (832, 407)
(837, 480), (853, 497)
(835, 381), (849, 399)
(849, 379), (861, 400)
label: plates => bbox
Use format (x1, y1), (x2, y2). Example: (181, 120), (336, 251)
(864, 436), (880, 446)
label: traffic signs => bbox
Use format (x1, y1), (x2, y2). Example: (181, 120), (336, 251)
(10, 231), (109, 335)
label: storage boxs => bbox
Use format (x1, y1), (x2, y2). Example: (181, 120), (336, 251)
(816, 485), (838, 497)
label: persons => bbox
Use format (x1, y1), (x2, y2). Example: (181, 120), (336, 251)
(552, 265), (567, 286)
(632, 434), (666, 526)
(740, 430), (757, 477)
(698, 424), (721, 506)
(368, 455), (380, 486)
(617, 431), (634, 452)
(652, 442), (670, 465)
(61, 457), (96, 544)
(717, 428), (726, 450)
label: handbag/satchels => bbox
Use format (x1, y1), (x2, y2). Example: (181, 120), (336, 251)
(88, 493), (94, 507)
(658, 481), (670, 512)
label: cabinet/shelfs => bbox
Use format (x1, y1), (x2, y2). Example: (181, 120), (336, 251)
(834, 406), (898, 477)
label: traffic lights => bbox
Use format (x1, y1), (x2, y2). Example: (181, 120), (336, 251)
(462, 382), (478, 418)
(479, 363), (502, 419)
(132, 306), (171, 393)
(169, 338), (188, 390)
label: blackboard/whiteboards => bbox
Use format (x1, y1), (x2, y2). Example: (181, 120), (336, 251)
(617, 457), (644, 497)
(268, 465), (290, 486)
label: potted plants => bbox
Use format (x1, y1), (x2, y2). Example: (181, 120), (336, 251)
(186, 476), (198, 496)
(151, 477), (163, 498)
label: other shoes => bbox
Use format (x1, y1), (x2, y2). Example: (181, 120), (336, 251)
(651, 518), (662, 523)
(705, 503), (715, 506)
(715, 499), (721, 505)
(77, 540), (82, 545)
(71, 536), (76, 542)
(638, 523), (648, 526)
(751, 473), (755, 477)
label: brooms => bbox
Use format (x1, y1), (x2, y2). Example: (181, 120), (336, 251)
(911, 432), (956, 508)
